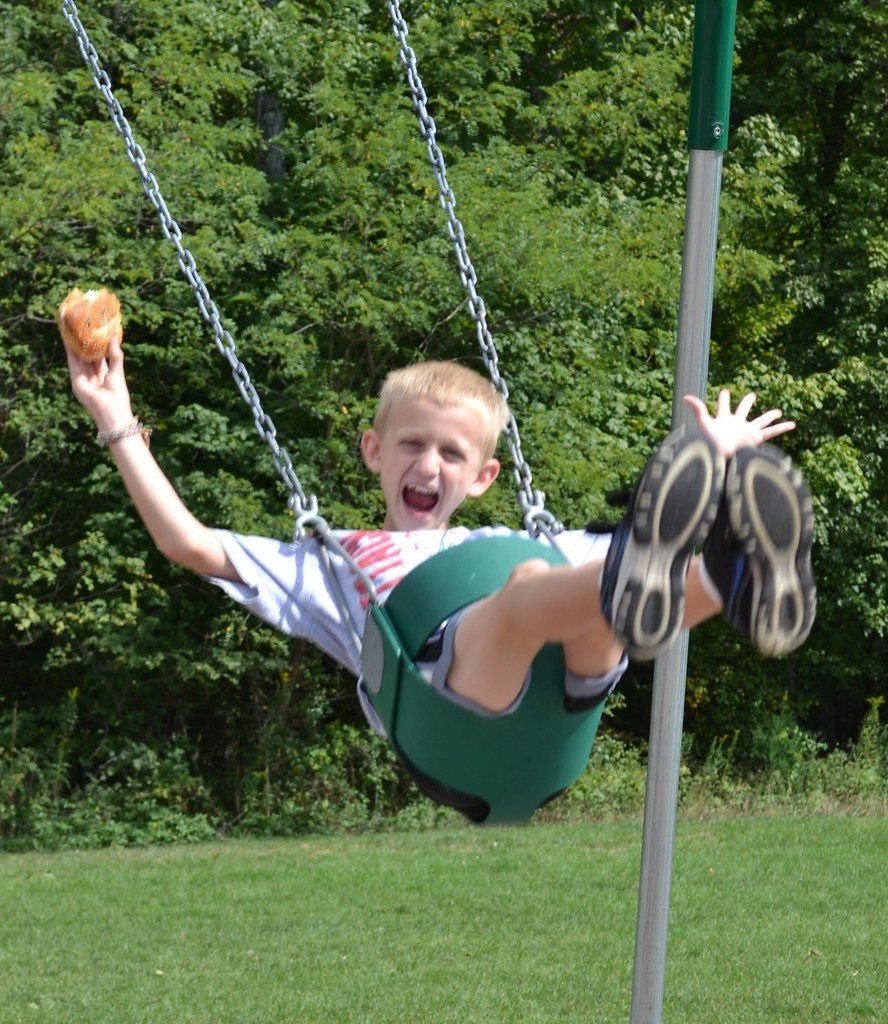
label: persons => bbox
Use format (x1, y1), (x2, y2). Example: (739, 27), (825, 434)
(65, 337), (817, 828)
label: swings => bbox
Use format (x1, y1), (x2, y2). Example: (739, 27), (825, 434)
(62, 1), (608, 831)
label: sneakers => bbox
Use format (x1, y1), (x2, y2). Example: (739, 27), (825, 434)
(585, 423), (726, 661)
(703, 444), (816, 657)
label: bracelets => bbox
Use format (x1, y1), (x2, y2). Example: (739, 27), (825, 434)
(99, 417), (152, 447)
